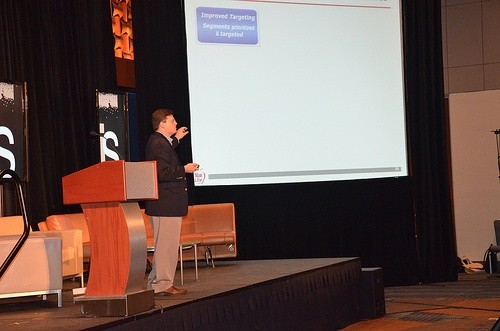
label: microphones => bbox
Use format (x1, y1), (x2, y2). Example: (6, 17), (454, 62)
(91, 131), (124, 160)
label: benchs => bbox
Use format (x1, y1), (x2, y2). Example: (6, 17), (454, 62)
(0, 203), (236, 307)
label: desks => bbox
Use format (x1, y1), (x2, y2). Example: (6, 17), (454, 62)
(146, 240), (201, 287)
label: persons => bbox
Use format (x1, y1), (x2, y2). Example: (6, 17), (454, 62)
(144, 109), (200, 297)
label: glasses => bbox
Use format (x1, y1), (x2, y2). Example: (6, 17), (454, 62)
(161, 118), (176, 122)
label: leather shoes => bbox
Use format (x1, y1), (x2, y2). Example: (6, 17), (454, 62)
(154, 285), (187, 295)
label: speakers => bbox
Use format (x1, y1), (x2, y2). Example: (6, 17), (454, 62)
(362, 267), (386, 319)
